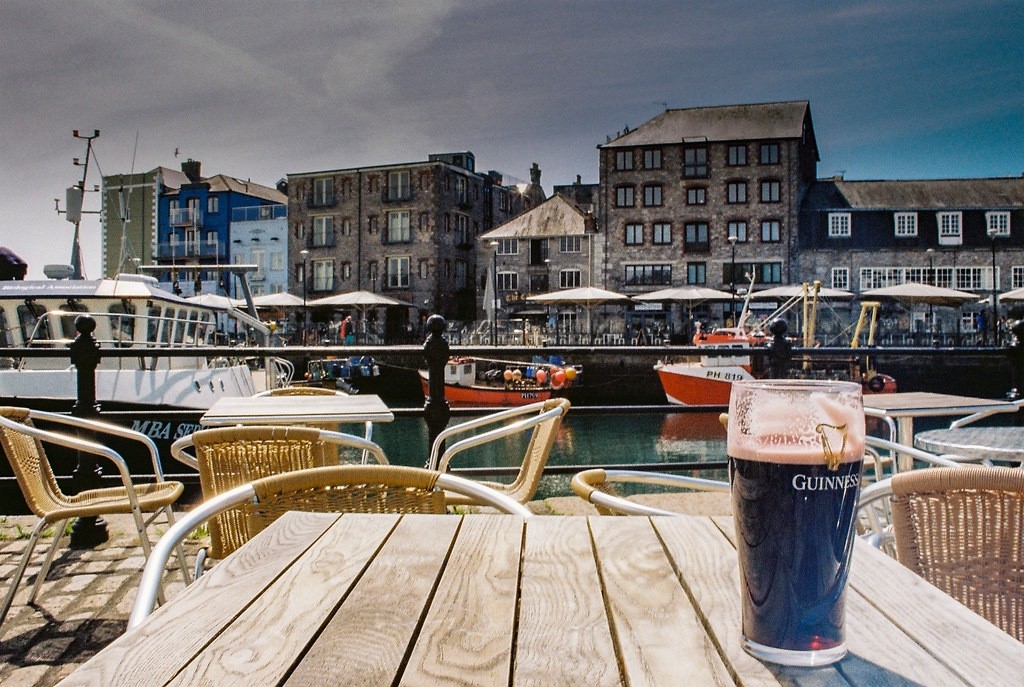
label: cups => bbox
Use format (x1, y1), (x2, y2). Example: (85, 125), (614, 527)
(726, 379), (867, 668)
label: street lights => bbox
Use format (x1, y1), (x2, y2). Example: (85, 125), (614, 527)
(544, 258), (554, 344)
(728, 236), (739, 330)
(132, 256), (141, 277)
(299, 248), (312, 379)
(924, 249), (935, 344)
(987, 226), (1001, 345)
(487, 240), (502, 345)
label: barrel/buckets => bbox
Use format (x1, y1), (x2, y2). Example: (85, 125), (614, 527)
(360, 365), (380, 377)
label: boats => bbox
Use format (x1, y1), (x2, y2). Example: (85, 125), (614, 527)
(306, 353), (377, 391)
(416, 355), (585, 411)
(650, 266), (900, 408)
(0, 126), (291, 501)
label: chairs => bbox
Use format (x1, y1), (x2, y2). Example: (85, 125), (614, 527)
(0, 406), (192, 629)
(125, 387), (1024, 644)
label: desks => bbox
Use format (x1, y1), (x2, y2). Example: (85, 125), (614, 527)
(862, 391), (1019, 473)
(200, 396), (395, 463)
(914, 427), (1024, 462)
(54, 510), (1024, 687)
